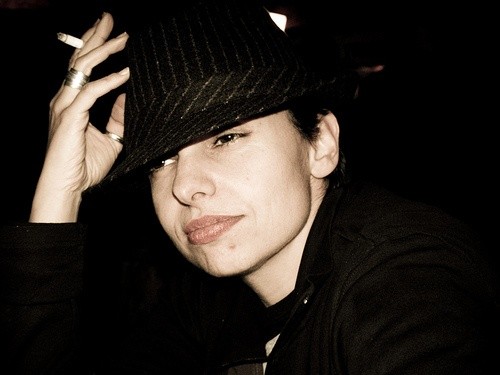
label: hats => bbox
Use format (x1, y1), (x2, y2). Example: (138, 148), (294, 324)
(110, 2), (337, 176)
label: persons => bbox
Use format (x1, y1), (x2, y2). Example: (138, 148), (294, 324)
(0, 0), (500, 374)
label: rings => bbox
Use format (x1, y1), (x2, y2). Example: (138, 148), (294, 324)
(63, 65), (89, 91)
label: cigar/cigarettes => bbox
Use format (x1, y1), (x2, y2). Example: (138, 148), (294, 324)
(53, 29), (84, 50)
